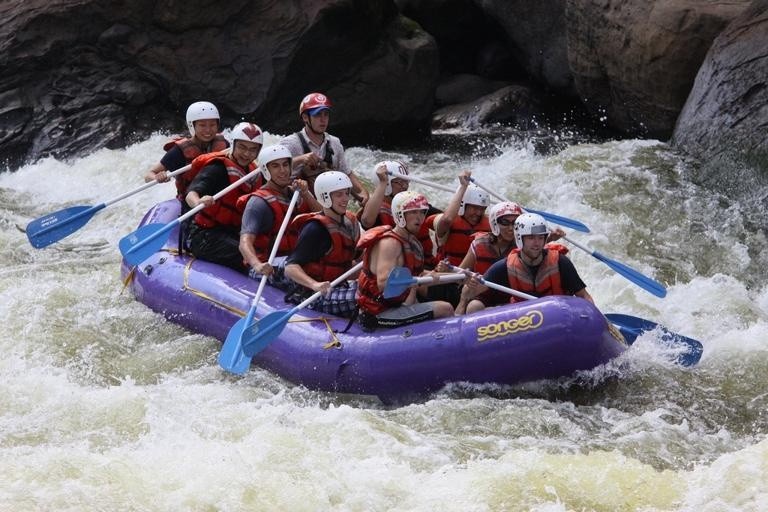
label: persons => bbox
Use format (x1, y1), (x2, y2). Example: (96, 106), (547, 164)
(459, 212), (595, 315)
(355, 158), (443, 271)
(431, 170), (567, 309)
(434, 201), (568, 315)
(141, 102), (235, 253)
(182, 121), (270, 277)
(352, 189), (473, 330)
(281, 170), (367, 322)
(235, 144), (324, 294)
(277, 91), (370, 208)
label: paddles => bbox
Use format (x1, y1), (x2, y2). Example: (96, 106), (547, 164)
(240, 258), (365, 359)
(26, 157), (211, 250)
(443, 261), (703, 369)
(117, 168), (260, 267)
(217, 189), (307, 375)
(465, 175), (667, 298)
(382, 266), (471, 299)
(385, 169), (591, 235)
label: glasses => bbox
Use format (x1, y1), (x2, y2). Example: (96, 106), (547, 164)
(499, 218), (516, 226)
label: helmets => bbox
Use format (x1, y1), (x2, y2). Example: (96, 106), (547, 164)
(488, 201), (524, 236)
(391, 191), (429, 228)
(230, 122), (263, 157)
(258, 145), (293, 181)
(373, 160), (408, 197)
(459, 186), (491, 217)
(185, 101), (221, 137)
(513, 213), (549, 250)
(300, 93), (333, 113)
(314, 171), (353, 209)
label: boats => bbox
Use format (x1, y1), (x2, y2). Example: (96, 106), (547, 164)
(118, 196), (628, 393)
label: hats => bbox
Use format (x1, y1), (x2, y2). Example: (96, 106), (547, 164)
(310, 107), (332, 115)
